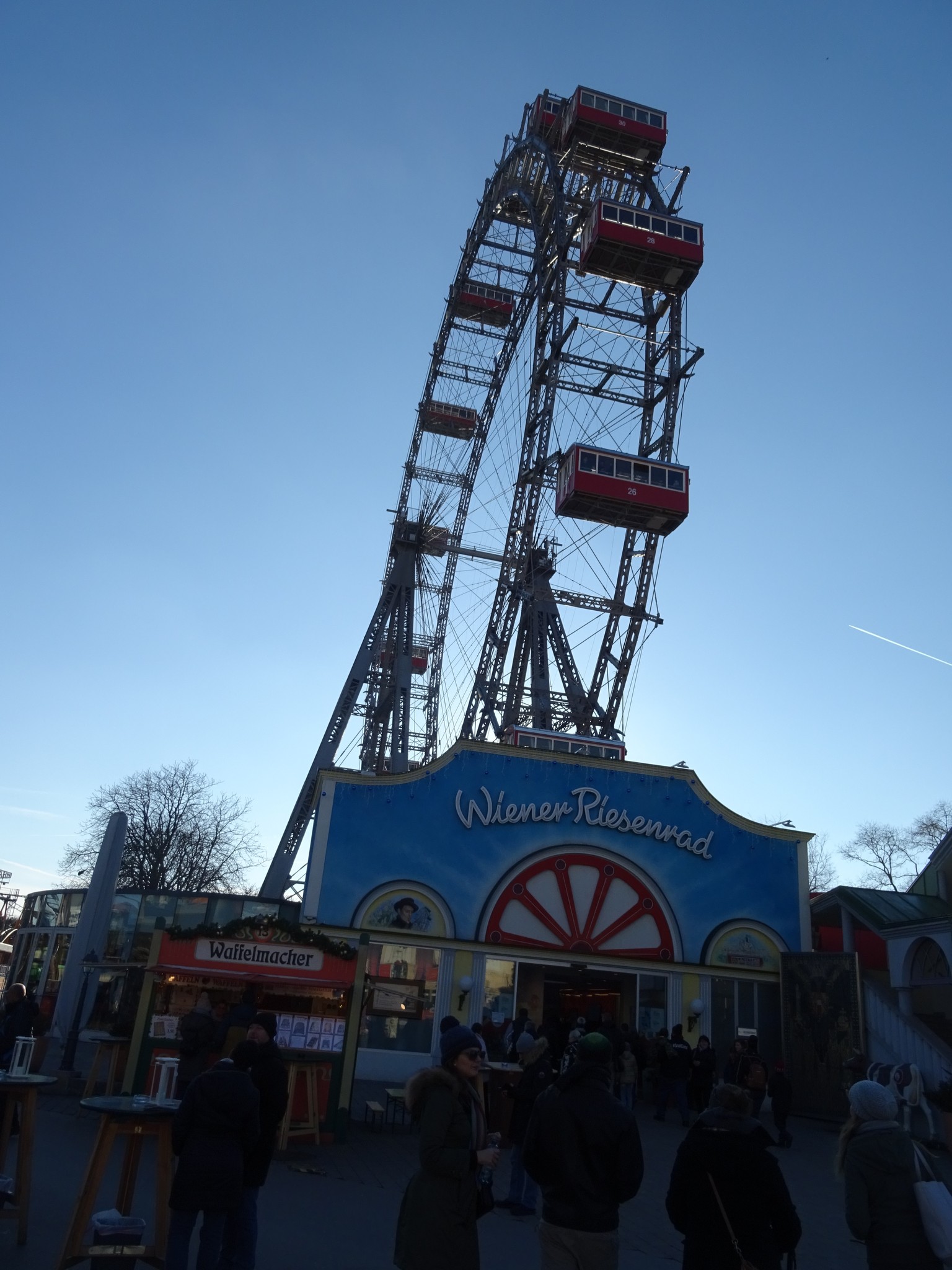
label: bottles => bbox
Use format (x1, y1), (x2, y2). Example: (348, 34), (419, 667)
(476, 1139), (500, 1185)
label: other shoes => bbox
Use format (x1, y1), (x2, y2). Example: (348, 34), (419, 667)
(495, 1195), (518, 1210)
(779, 1133), (793, 1147)
(512, 1203), (536, 1217)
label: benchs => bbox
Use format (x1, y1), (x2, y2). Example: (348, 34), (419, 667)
(366, 1101), (384, 1124)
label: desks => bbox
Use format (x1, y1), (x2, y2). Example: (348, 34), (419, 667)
(488, 1061), (522, 1072)
(61, 1096), (179, 1270)
(83, 1036), (131, 1098)
(0, 1073), (59, 1245)
(386, 1089), (406, 1122)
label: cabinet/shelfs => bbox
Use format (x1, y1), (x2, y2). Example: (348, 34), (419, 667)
(10, 1035), (38, 1073)
(150, 1056), (180, 1098)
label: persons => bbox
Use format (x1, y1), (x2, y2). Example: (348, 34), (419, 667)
(163, 1010), (290, 1270)
(664, 1084), (802, 1270)
(367, 987), (794, 1215)
(0, 983), (256, 1136)
(393, 1029), (502, 1270)
(516, 1032), (644, 1270)
(836, 1080), (952, 1270)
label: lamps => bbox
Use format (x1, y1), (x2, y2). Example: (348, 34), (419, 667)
(475, 734), (488, 741)
(688, 998), (705, 1031)
(362, 770), (376, 777)
(770, 819), (795, 828)
(456, 977), (472, 1010)
(578, 746), (592, 754)
(670, 760), (689, 768)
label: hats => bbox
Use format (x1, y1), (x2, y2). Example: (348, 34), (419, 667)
(250, 1012), (277, 1037)
(848, 1080), (897, 1119)
(672, 1024), (683, 1032)
(516, 1031), (534, 1052)
(580, 1032), (609, 1049)
(197, 992), (212, 1012)
(774, 1059), (786, 1072)
(439, 1015), (483, 1070)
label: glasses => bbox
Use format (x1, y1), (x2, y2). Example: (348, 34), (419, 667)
(462, 1049), (486, 1061)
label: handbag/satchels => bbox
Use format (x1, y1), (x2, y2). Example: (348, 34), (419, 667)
(910, 1142), (952, 1259)
(474, 1175), (495, 1221)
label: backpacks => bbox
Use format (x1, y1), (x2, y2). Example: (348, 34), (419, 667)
(745, 1055), (768, 1088)
